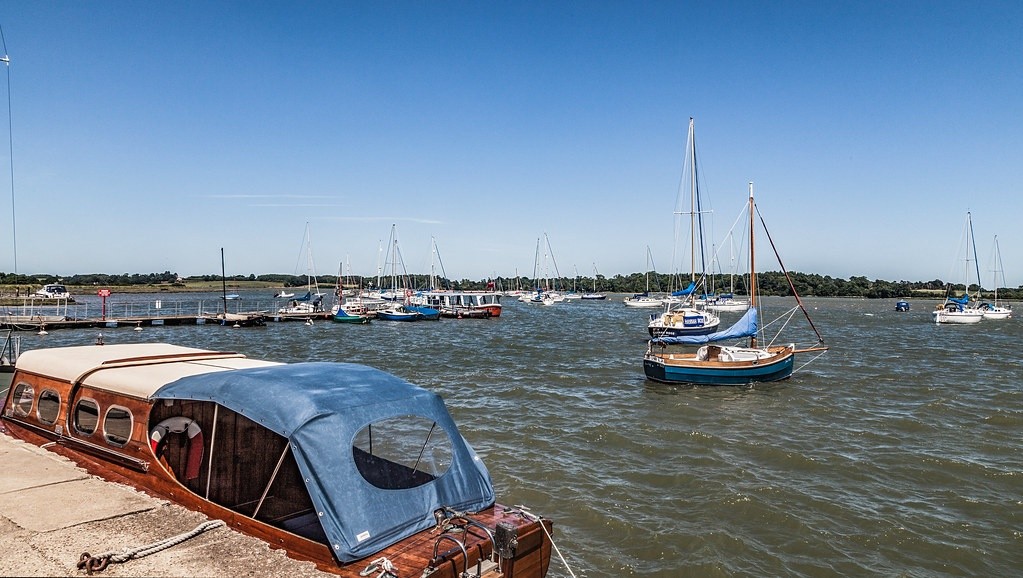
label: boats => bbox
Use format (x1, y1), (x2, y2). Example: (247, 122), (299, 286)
(647, 116), (721, 339)
(0, 332), (555, 578)
(895, 299), (909, 312)
(31, 284), (74, 301)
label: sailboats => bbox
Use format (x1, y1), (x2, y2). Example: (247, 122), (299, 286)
(643, 181), (830, 386)
(220, 220), (504, 324)
(505, 233), (607, 306)
(622, 231), (751, 312)
(932, 208), (1013, 324)
